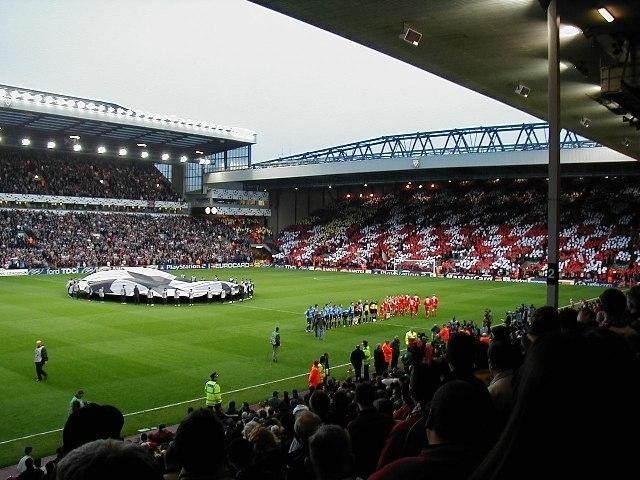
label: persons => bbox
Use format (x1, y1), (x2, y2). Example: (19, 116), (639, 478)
(0, 145), (639, 479)
